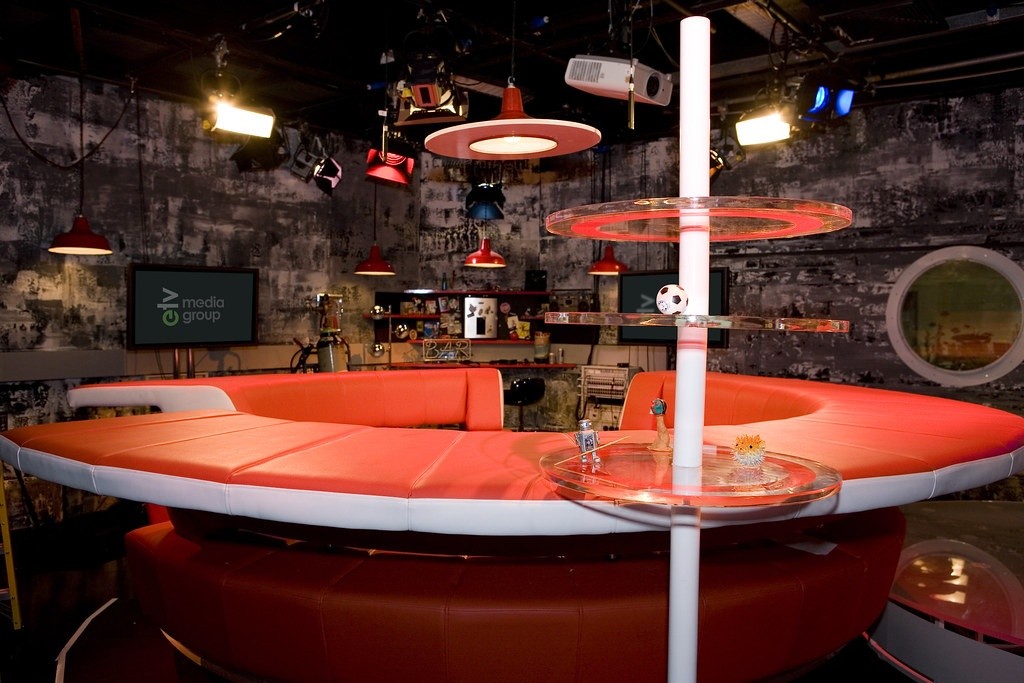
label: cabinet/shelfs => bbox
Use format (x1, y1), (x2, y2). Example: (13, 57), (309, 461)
(362, 290), (557, 346)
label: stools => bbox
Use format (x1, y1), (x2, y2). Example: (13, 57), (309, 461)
(503, 377), (545, 430)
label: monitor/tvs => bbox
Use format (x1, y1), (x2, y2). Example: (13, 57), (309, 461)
(620, 267), (730, 350)
(126, 261), (260, 353)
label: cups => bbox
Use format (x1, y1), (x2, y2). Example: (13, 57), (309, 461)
(534, 331), (550, 364)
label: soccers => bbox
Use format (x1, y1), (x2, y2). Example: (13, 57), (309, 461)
(657, 284), (690, 313)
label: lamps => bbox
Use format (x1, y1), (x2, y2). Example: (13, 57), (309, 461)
(425, 13), (602, 162)
(352, 128), (415, 275)
(464, 180), (508, 269)
(290, 121), (344, 197)
(710, 104), (745, 186)
(48, 0), (113, 256)
(587, 142), (630, 277)
(198, 67), (289, 173)
(734, 18), (857, 147)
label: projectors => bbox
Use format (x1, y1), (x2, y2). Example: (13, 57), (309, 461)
(565, 54), (672, 106)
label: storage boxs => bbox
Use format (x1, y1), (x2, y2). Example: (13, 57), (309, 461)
(504, 311), (520, 341)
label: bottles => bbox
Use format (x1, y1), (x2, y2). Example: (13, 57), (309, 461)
(557, 348), (563, 364)
(464, 297), (497, 339)
(548, 352), (556, 365)
